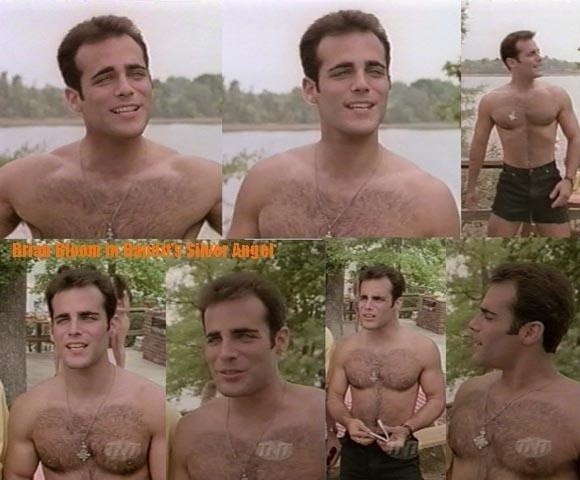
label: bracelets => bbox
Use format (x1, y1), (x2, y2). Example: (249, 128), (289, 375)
(400, 423), (413, 439)
(327, 427), (338, 434)
(563, 176), (575, 189)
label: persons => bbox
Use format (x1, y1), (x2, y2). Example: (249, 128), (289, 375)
(325, 326), (342, 467)
(326, 262), (446, 480)
(225, 9), (460, 239)
(0, 14), (222, 240)
(447, 261), (580, 480)
(464, 29), (580, 237)
(0, 264), (167, 480)
(169, 270), (325, 480)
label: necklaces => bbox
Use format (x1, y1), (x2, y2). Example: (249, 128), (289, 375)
(472, 368), (557, 451)
(226, 381), (286, 480)
(358, 329), (399, 383)
(508, 98), (531, 120)
(313, 141), (383, 238)
(79, 133), (146, 239)
